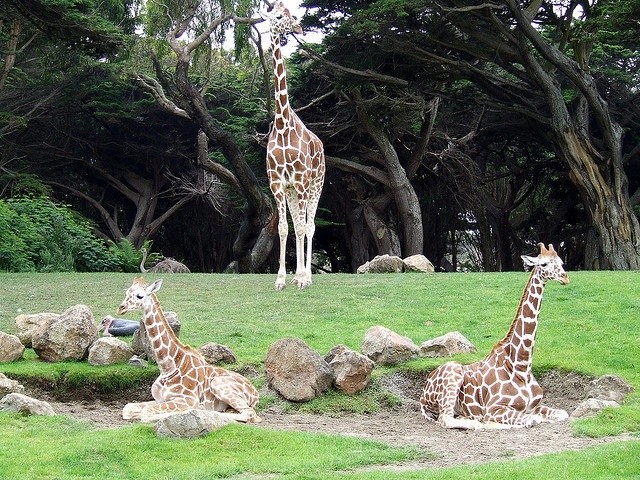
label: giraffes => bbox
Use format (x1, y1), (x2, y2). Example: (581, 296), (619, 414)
(257, 1), (325, 291)
(116, 277), (262, 424)
(419, 242), (570, 429)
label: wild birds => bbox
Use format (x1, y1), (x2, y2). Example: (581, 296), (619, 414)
(101, 315), (114, 337)
